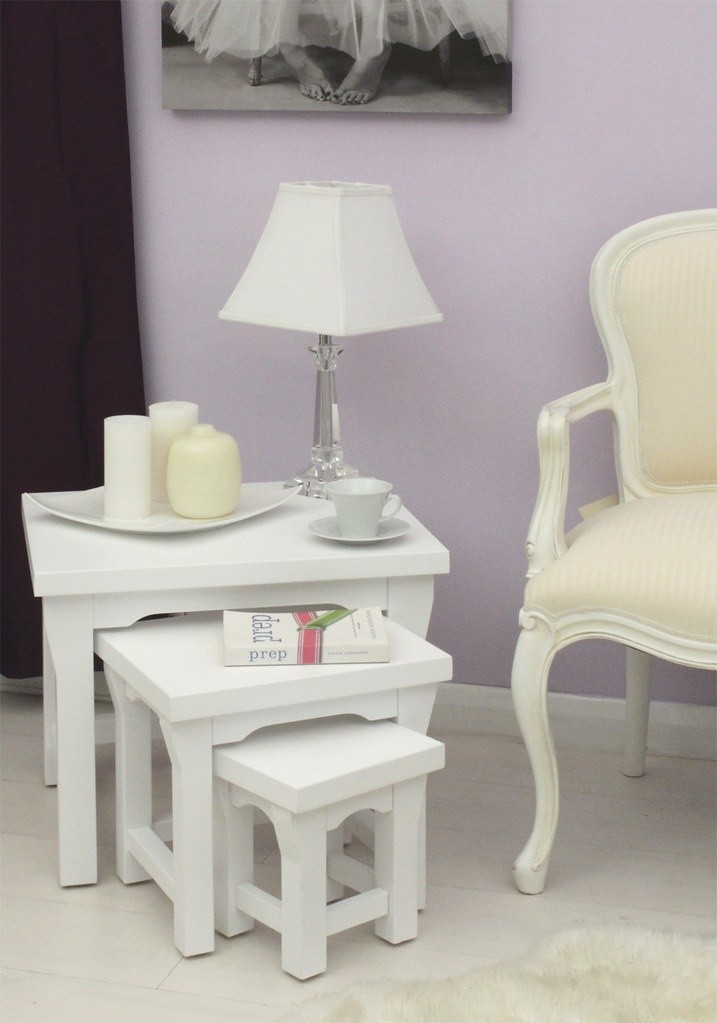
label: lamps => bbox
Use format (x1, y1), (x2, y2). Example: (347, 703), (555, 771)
(216, 182), (448, 497)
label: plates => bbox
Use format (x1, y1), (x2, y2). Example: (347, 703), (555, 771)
(309, 517), (411, 544)
(25, 482), (303, 535)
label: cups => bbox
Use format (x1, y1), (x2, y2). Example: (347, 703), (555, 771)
(326, 478), (401, 538)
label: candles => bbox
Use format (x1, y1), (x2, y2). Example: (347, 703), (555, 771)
(103, 414), (152, 518)
(149, 400), (202, 505)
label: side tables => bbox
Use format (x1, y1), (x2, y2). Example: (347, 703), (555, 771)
(91, 611), (456, 961)
(16, 482), (455, 890)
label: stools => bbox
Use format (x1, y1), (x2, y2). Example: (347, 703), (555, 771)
(214, 712), (449, 983)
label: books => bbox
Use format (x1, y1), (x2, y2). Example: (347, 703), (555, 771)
(221, 606), (390, 666)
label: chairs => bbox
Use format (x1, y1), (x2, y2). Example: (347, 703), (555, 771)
(509, 209), (717, 893)
